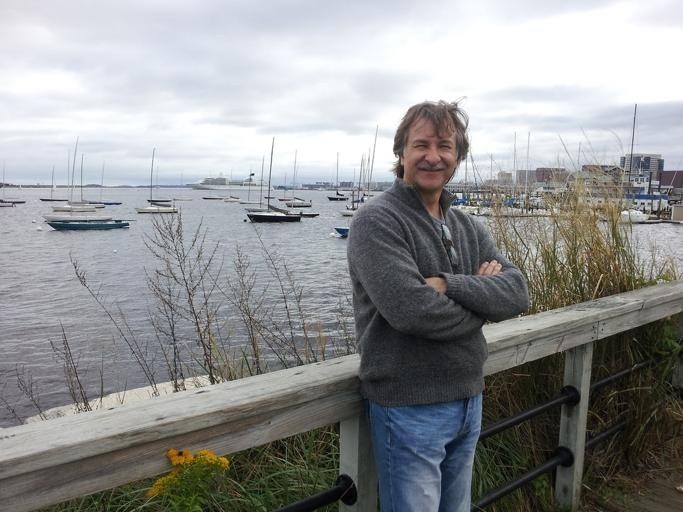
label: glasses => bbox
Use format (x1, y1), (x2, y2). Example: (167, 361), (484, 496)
(440, 223), (463, 276)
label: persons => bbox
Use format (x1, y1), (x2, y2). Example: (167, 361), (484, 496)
(345, 95), (532, 511)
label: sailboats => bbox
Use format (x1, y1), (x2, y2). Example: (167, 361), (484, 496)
(566, 102), (670, 224)
(454, 104), (562, 216)
(0, 160), (28, 208)
